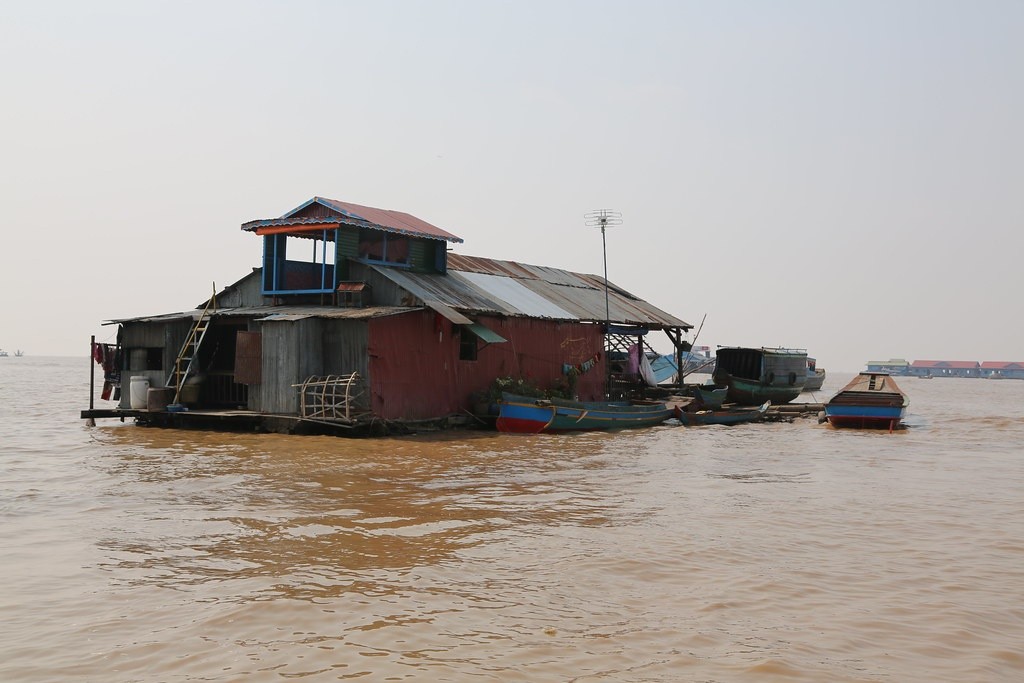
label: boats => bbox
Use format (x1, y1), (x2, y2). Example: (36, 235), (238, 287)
(803, 357), (826, 391)
(645, 351), (708, 386)
(493, 391), (697, 436)
(672, 398), (773, 428)
(821, 369), (910, 435)
(710, 341), (809, 405)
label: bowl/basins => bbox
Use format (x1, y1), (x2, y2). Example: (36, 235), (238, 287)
(167, 405), (183, 411)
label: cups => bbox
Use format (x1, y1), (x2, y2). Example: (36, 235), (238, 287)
(185, 408), (188, 412)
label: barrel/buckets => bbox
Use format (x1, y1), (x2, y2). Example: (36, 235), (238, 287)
(146, 387), (177, 413)
(179, 385), (199, 402)
(129, 375), (150, 409)
